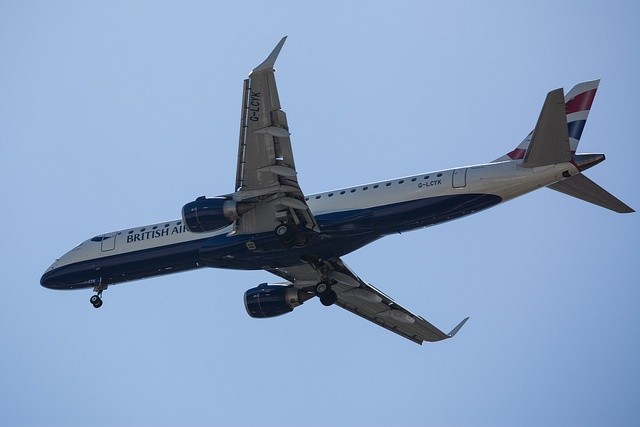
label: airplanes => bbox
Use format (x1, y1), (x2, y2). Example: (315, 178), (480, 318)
(38, 34), (636, 347)
